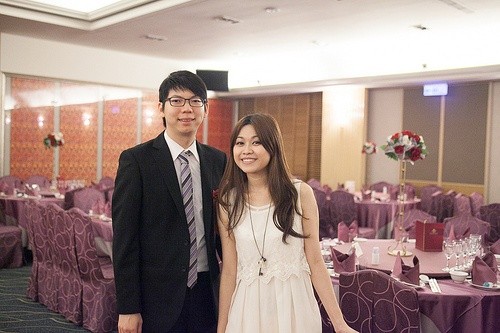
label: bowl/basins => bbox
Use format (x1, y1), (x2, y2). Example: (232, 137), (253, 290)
(449, 271), (469, 283)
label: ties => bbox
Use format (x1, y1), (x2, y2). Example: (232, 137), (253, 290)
(177, 151), (198, 289)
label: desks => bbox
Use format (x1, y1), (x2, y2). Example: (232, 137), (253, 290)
(88, 213), (113, 260)
(0, 194), (65, 249)
(356, 197), (422, 232)
(320, 238), (500, 333)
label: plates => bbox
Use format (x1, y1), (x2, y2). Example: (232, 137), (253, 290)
(468, 279), (500, 291)
(320, 238), (340, 278)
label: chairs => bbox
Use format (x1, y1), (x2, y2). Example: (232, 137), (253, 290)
(0, 175), (117, 333)
(307, 179), (500, 333)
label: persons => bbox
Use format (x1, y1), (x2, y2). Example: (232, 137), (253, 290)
(215, 113), (361, 333)
(112, 71), (226, 333)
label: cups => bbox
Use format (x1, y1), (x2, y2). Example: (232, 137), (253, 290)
(322, 237), (331, 255)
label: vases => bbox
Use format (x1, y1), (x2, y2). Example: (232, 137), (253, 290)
(387, 160), (412, 257)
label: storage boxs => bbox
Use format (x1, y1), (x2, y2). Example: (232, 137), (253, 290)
(415, 219), (445, 251)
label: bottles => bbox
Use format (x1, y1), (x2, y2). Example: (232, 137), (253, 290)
(371, 247), (379, 265)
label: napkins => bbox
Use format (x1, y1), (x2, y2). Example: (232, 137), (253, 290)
(490, 239), (500, 254)
(329, 245), (356, 274)
(472, 250), (497, 284)
(338, 219), (358, 242)
(447, 224), (472, 239)
(393, 251), (419, 285)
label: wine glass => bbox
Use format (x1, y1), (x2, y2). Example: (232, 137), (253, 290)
(442, 234), (481, 271)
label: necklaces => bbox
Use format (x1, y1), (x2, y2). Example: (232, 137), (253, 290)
(247, 192), (272, 276)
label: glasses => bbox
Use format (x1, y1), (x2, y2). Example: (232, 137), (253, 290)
(162, 95), (207, 107)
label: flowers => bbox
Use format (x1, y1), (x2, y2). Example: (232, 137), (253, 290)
(361, 130), (429, 167)
(44, 133), (64, 150)
